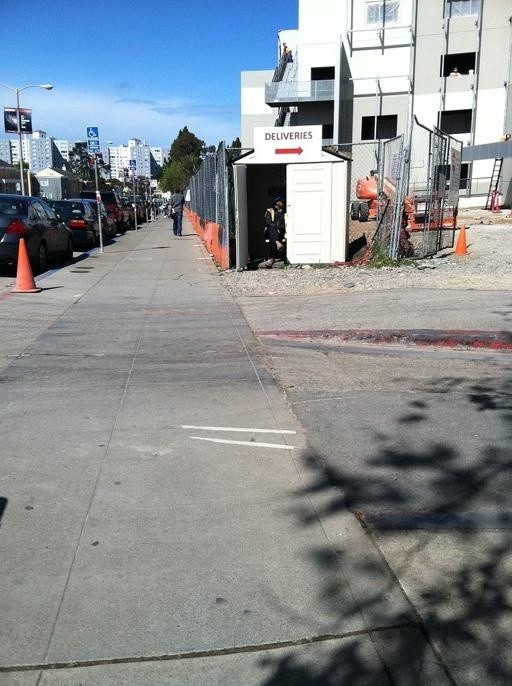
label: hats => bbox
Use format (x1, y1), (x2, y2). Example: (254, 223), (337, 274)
(272, 193), (286, 202)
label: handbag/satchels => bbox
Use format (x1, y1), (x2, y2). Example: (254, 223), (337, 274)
(171, 208), (176, 215)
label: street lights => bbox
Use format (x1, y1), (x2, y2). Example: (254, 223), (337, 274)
(1, 84), (55, 196)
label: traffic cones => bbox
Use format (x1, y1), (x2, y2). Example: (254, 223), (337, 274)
(451, 223), (470, 258)
(10, 237), (43, 294)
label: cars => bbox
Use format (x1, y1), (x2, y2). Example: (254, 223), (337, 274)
(0, 188), (160, 273)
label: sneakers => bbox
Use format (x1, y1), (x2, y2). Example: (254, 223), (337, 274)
(265, 257), (274, 267)
(277, 243), (284, 253)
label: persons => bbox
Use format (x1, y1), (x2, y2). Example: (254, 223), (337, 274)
(170, 187), (186, 236)
(264, 192), (287, 267)
(159, 196), (174, 220)
(283, 42), (289, 62)
(449, 66), (462, 75)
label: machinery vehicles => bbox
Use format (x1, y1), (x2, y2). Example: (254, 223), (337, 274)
(350, 169), (459, 232)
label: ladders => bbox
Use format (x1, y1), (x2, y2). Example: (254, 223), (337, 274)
(484, 157), (503, 210)
